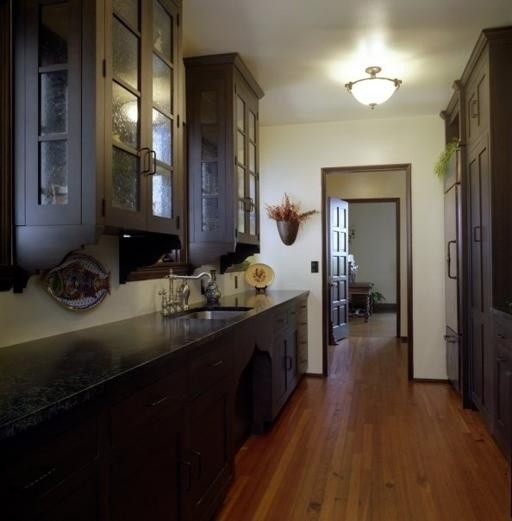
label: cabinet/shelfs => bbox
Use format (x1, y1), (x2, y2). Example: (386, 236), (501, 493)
(180, 53), (263, 275)
(1, 389), (101, 521)
(438, 24), (512, 470)
(270, 300), (295, 400)
(16, 0), (179, 292)
(102, 330), (238, 521)
(296, 296), (308, 377)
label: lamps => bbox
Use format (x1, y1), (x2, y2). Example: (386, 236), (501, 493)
(342, 64), (402, 110)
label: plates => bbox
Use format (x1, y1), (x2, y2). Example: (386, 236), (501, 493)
(245, 263), (274, 288)
(43, 255), (111, 313)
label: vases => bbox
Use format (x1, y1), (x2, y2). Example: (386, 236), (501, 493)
(277, 218), (299, 245)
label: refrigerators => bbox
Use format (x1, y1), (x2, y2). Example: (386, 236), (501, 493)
(443, 149), (465, 396)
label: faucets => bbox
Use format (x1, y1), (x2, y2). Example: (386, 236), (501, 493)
(168, 269), (214, 313)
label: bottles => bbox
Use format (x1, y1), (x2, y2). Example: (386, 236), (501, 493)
(205, 270), (221, 303)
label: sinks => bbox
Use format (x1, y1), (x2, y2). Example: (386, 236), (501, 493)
(164, 310), (246, 320)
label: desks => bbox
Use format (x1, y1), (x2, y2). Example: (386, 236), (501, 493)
(348, 281), (374, 323)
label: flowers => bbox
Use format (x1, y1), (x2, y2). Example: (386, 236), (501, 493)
(264, 194), (318, 222)
